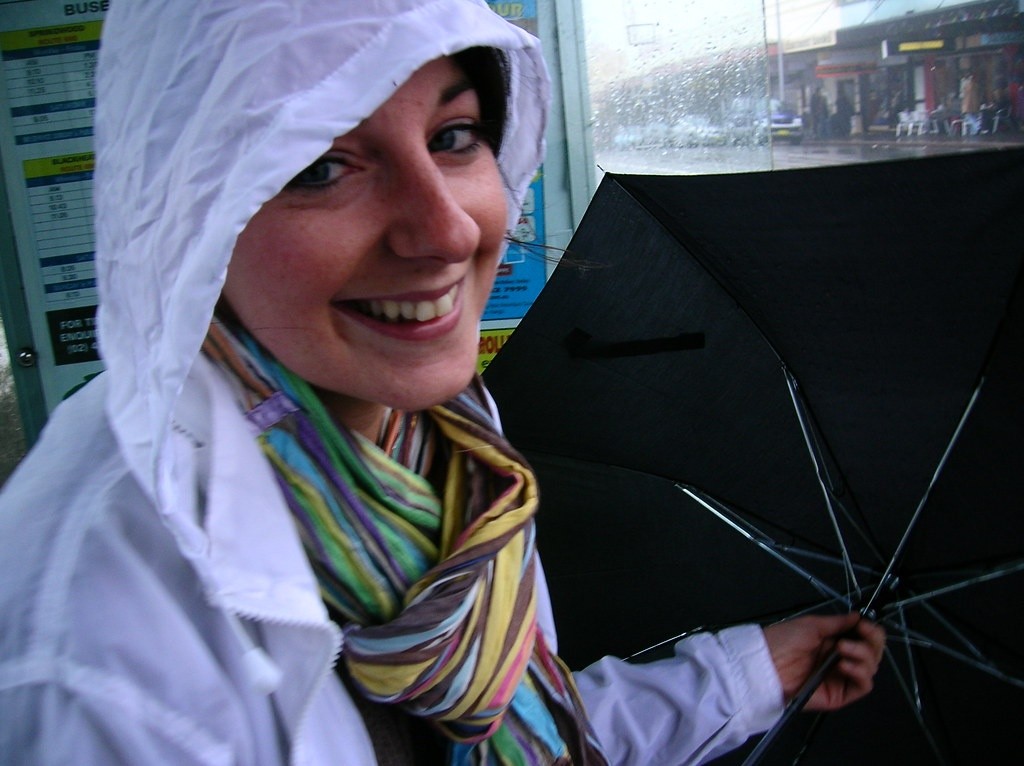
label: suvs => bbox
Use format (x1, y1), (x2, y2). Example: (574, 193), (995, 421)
(730, 98), (803, 147)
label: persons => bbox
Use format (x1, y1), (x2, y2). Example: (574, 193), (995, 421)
(929, 72), (1024, 137)
(1, 0), (887, 764)
(810, 86), (855, 140)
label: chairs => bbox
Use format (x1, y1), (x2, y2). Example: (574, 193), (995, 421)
(991, 96), (1013, 132)
(895, 110), (928, 137)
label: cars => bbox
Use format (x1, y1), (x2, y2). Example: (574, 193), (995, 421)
(671, 116), (725, 145)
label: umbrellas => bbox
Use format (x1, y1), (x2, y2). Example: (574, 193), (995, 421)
(482, 148), (1023, 766)
